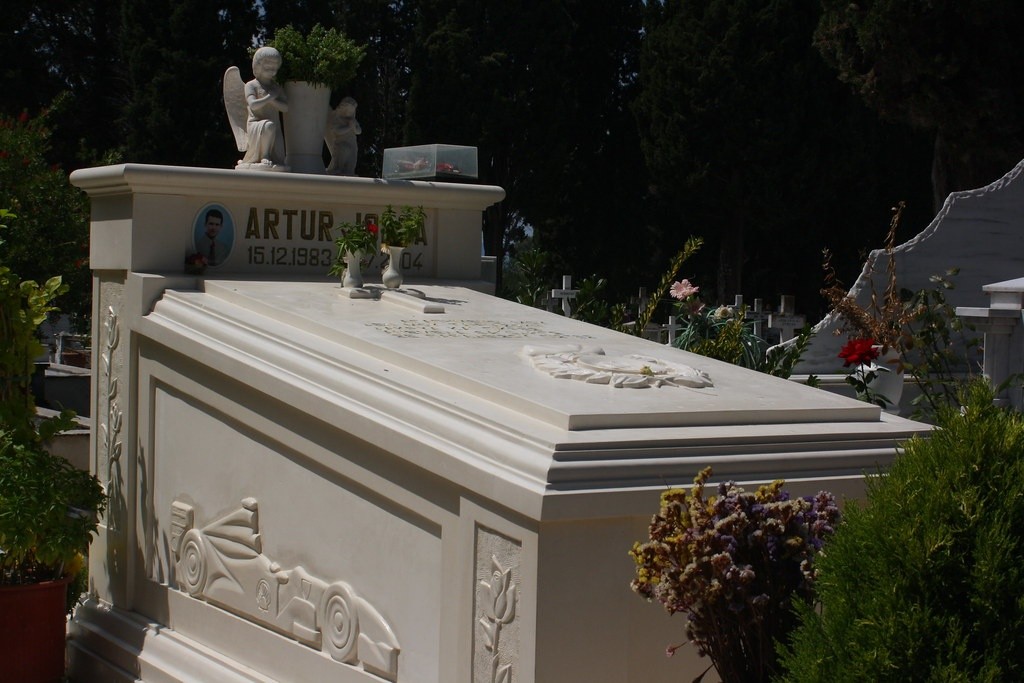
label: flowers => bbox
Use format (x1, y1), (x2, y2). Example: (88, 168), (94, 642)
(628, 471), (842, 683)
(840, 336), (888, 407)
(327, 219), (378, 277)
(668, 280), (749, 361)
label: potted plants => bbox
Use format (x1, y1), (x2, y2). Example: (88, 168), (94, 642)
(821, 200), (930, 415)
(378, 203), (427, 288)
(0, 435), (111, 683)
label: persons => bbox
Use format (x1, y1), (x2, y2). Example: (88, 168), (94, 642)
(240, 47), (290, 167)
(196, 209), (230, 267)
(325, 96), (361, 175)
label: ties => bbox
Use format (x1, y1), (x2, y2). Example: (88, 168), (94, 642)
(208, 241), (216, 266)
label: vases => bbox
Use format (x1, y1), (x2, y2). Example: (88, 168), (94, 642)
(341, 248), (364, 289)
